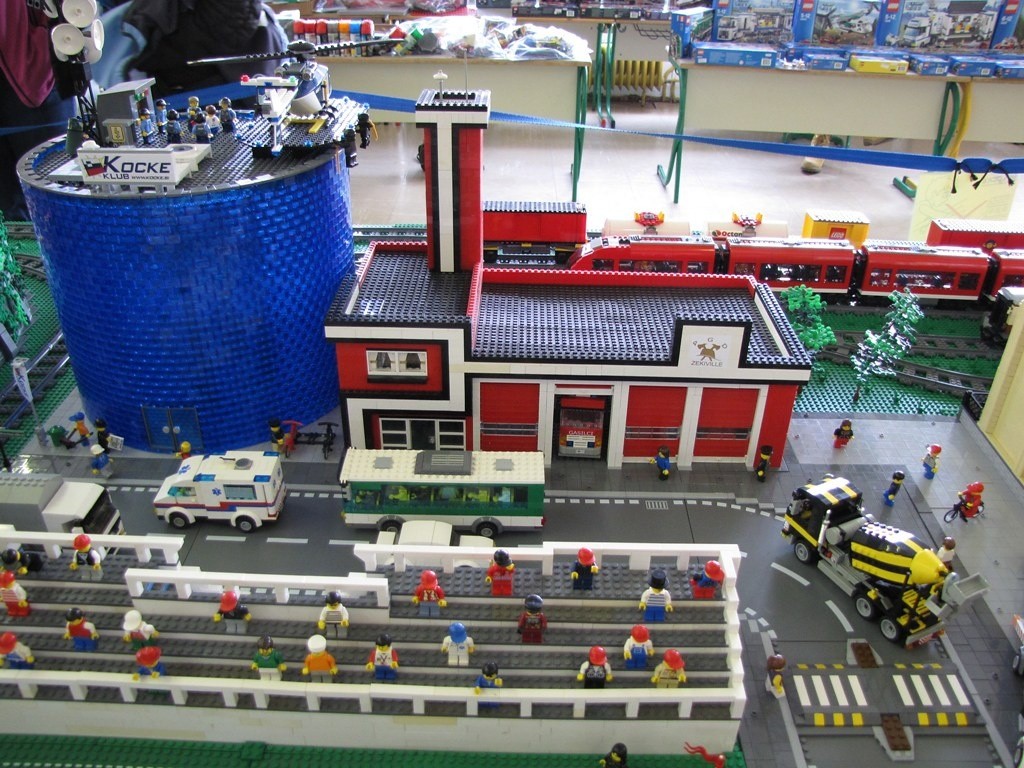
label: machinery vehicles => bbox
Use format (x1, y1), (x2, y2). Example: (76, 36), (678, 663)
(779, 472), (989, 650)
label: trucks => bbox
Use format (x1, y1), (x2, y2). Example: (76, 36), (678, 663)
(902, 10), (984, 49)
(0, 470), (126, 557)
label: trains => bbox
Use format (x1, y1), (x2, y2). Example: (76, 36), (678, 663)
(566, 235), (1024, 307)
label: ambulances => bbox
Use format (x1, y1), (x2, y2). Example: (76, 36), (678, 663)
(154, 448), (288, 532)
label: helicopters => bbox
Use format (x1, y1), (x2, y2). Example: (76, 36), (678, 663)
(185, 38), (400, 159)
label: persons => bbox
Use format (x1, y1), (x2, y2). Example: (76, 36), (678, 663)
(269, 417), (285, 453)
(175, 442), (192, 460)
(957, 482), (985, 518)
(936, 537), (956, 573)
(354, 483), (511, 519)
(69, 535), (103, 581)
(67, 411), (116, 478)
(649, 446), (671, 481)
(86, 0), (294, 108)
(757, 445), (775, 483)
(919, 444), (942, 480)
(832, 417), (857, 450)
(140, 95), (237, 146)
(0, 0), (86, 222)
(599, 742), (628, 768)
(881, 470), (905, 507)
(0, 548), (728, 696)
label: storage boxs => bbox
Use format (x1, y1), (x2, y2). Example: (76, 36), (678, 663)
(667, 0), (1024, 79)
(474, 0), (669, 21)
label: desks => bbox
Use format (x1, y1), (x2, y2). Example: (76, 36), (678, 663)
(506, 15), (672, 130)
(293, 55), (600, 204)
(965, 70), (1024, 151)
(654, 57), (972, 205)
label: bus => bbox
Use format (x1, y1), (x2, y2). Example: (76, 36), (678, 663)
(338, 445), (549, 538)
